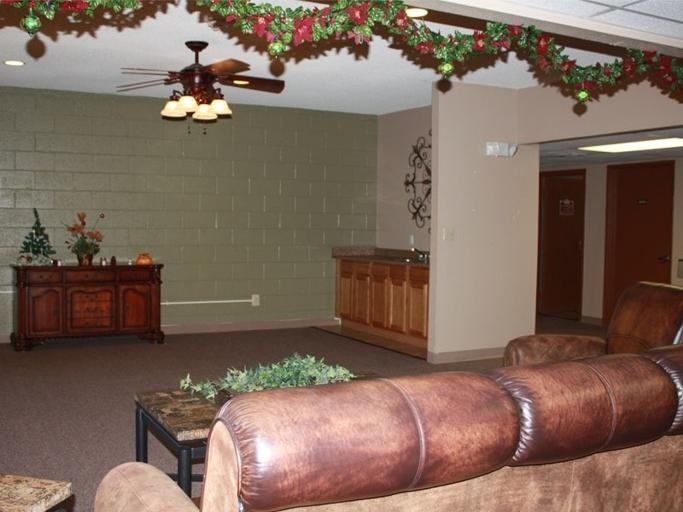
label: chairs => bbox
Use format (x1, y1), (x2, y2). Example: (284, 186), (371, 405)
(502, 279), (682, 368)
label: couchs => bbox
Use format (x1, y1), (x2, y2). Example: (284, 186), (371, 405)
(91, 346), (682, 511)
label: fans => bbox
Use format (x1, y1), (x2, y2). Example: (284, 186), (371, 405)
(113, 42), (289, 97)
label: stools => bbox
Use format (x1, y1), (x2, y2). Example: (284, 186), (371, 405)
(1, 473), (77, 510)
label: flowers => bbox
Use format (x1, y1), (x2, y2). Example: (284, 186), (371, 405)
(64, 206), (105, 256)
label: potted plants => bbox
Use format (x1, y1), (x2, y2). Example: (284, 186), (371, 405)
(19, 208), (57, 267)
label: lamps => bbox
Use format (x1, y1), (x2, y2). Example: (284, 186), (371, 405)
(161, 42), (233, 125)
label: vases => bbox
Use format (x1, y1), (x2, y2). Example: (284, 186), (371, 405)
(76, 253), (93, 267)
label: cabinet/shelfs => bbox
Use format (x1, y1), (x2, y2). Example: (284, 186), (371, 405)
(8, 258), (164, 351)
(331, 246), (428, 361)
